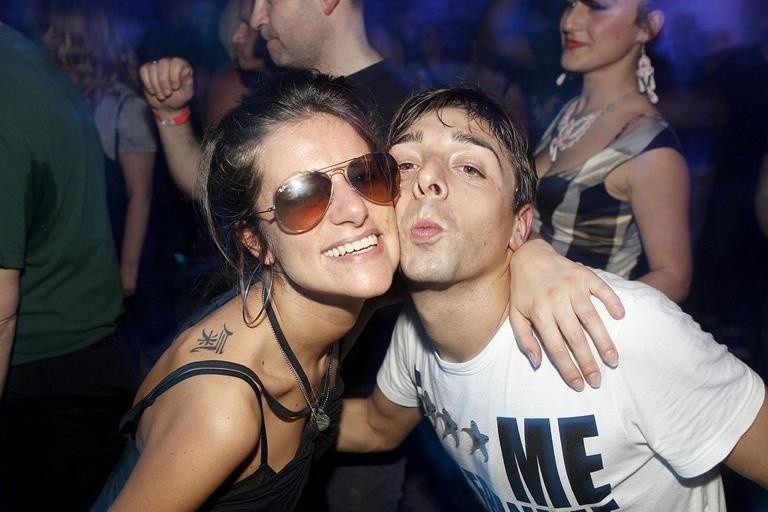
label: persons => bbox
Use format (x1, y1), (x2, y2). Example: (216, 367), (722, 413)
(0, 1), (422, 512)
(405, 1), (692, 302)
(337, 75), (768, 511)
(95, 66), (626, 512)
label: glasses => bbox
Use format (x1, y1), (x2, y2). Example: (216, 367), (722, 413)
(242, 151), (402, 235)
